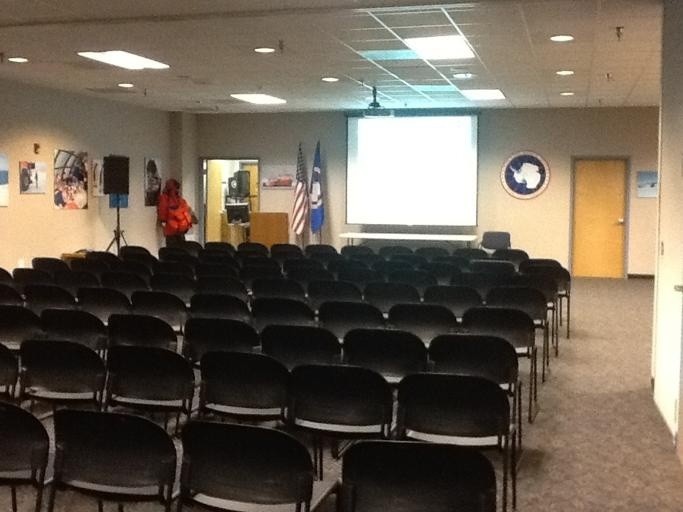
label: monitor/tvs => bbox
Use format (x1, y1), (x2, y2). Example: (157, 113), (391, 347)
(227, 170), (251, 197)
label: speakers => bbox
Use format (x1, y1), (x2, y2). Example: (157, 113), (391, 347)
(104, 155), (131, 195)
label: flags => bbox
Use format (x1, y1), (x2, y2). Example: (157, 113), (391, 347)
(308, 139), (324, 233)
(291, 140), (309, 237)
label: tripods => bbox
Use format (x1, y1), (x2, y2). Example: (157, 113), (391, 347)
(106, 196), (130, 253)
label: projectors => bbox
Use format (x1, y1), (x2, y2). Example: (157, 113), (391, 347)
(359, 106), (395, 117)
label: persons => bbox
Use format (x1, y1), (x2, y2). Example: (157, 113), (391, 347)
(157, 179), (193, 247)
